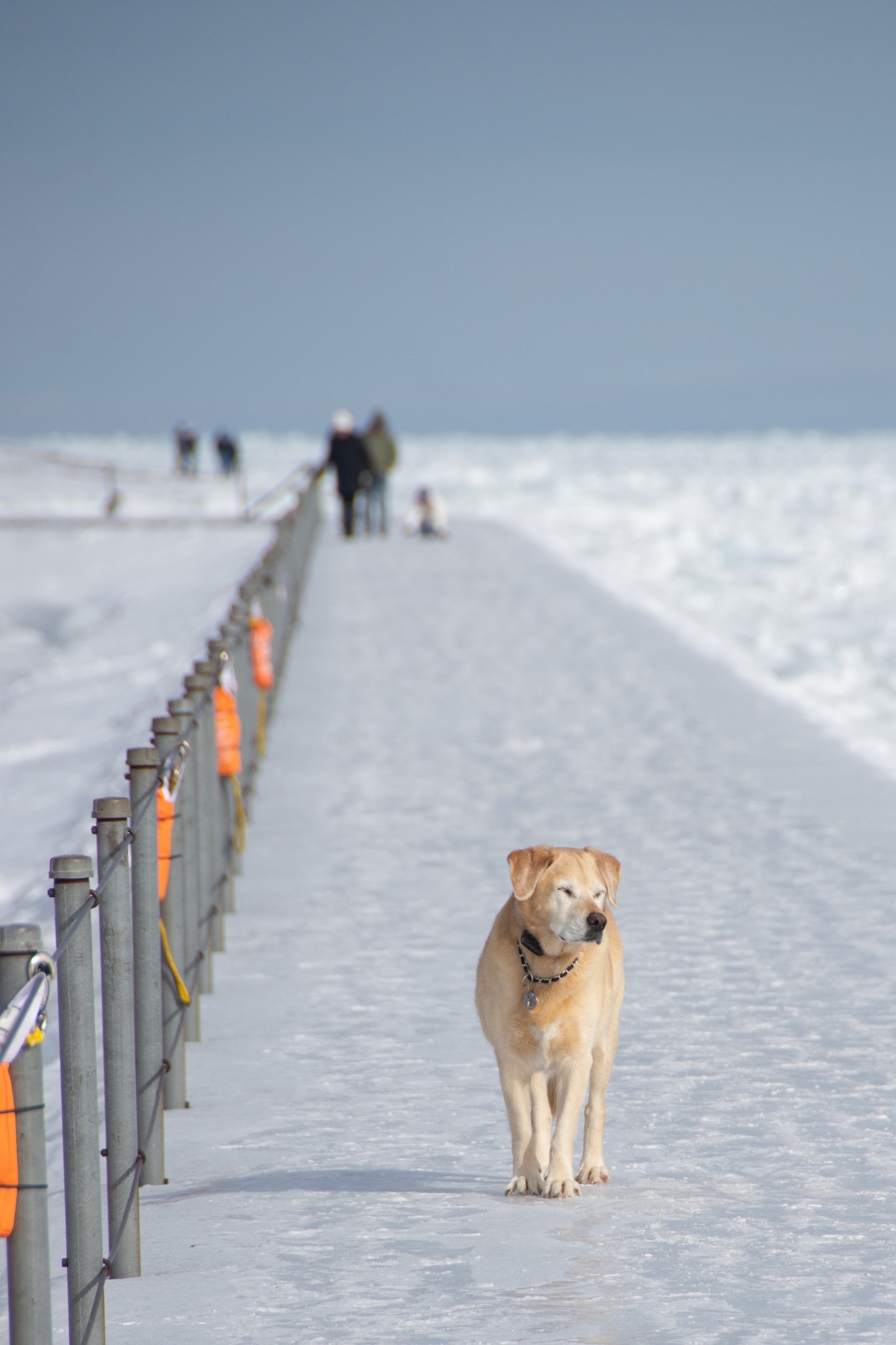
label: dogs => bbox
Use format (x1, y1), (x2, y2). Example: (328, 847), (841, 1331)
(473, 844), (627, 1197)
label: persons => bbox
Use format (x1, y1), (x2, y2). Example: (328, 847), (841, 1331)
(402, 480), (450, 536)
(173, 425), (194, 475)
(219, 434), (234, 475)
(313, 408), (371, 538)
(361, 406), (398, 537)
